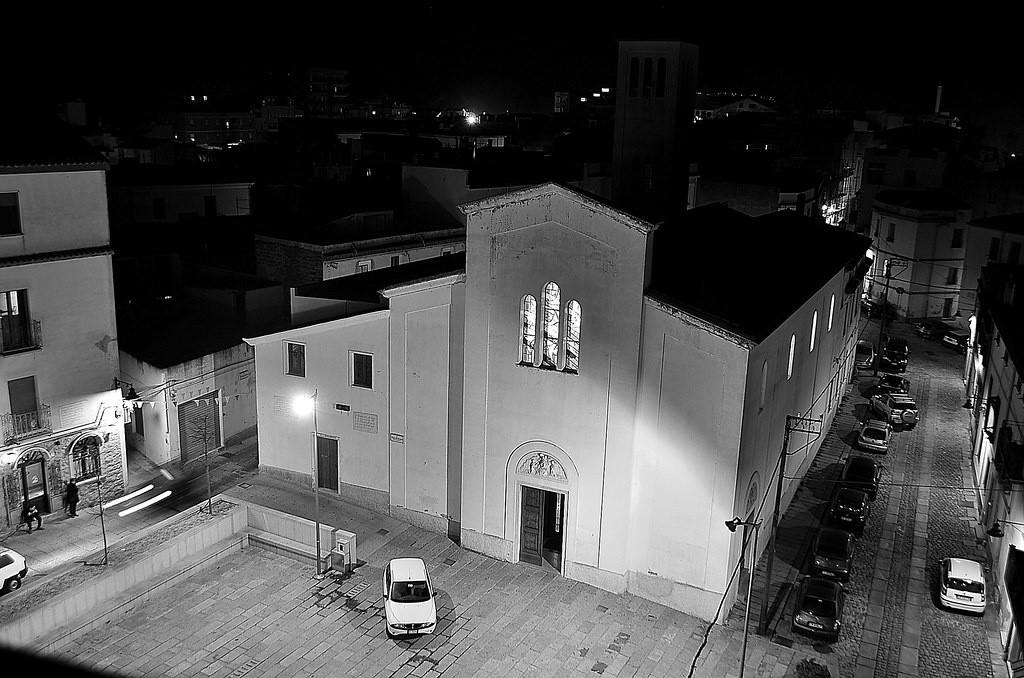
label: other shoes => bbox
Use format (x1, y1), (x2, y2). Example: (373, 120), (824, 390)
(74, 513), (79, 516)
(71, 514), (74, 518)
(37, 527), (45, 530)
(29, 529), (33, 534)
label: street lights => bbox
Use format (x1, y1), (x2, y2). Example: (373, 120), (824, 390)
(290, 387), (326, 581)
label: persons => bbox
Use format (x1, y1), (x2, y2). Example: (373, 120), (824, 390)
(22, 504), (45, 534)
(66, 478), (80, 518)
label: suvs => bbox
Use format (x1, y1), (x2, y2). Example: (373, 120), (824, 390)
(868, 393), (920, 430)
(381, 558), (438, 638)
(791, 575), (851, 643)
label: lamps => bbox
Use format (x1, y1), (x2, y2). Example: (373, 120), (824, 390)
(114, 376), (141, 402)
(986, 519), (1024, 538)
(962, 397), (988, 410)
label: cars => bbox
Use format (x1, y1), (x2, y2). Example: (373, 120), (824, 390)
(839, 454), (882, 501)
(876, 373), (911, 395)
(937, 555), (989, 617)
(808, 526), (857, 579)
(915, 320), (955, 341)
(0, 545), (29, 597)
(826, 486), (870, 535)
(884, 337), (912, 359)
(855, 419), (893, 455)
(941, 330), (970, 356)
(860, 298), (896, 321)
(855, 339), (878, 369)
(880, 351), (909, 373)
